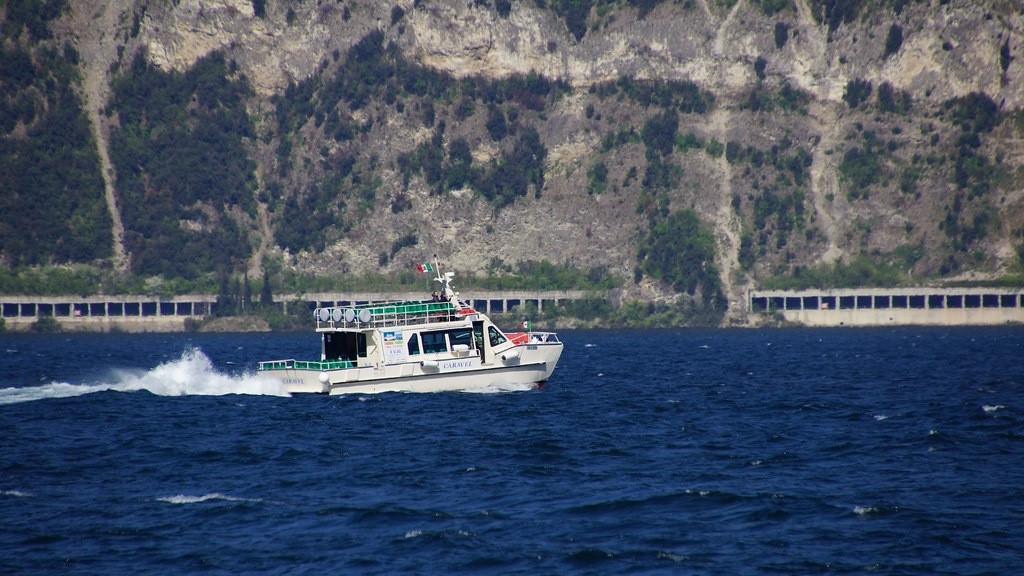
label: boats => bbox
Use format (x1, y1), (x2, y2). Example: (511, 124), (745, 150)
(254, 254), (564, 396)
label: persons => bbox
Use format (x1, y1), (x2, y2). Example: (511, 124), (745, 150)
(431, 290), (451, 322)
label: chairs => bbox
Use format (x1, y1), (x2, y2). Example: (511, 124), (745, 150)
(354, 301), (458, 326)
(297, 357), (353, 369)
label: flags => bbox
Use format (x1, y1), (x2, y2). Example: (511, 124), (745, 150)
(417, 262), (433, 272)
(521, 320), (531, 328)
